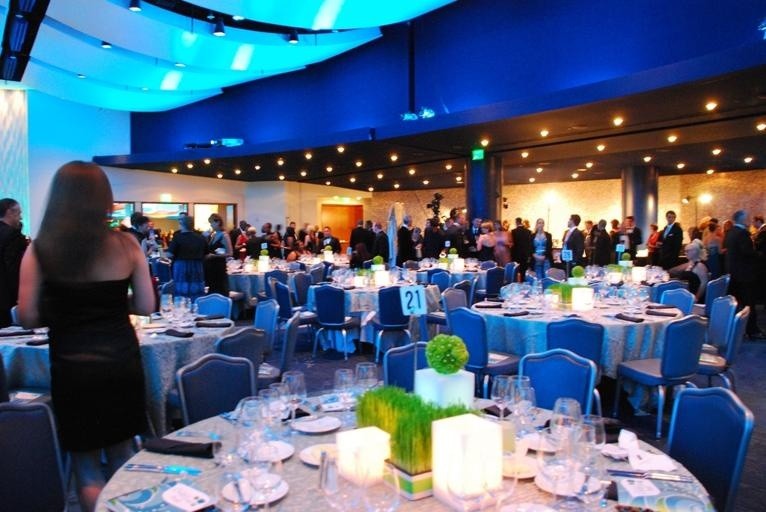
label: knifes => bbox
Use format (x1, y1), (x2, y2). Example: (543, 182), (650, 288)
(607, 469), (694, 484)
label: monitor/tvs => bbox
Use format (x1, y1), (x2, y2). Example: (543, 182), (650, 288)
(141, 202), (188, 239)
(193, 203), (235, 233)
(105, 201), (135, 232)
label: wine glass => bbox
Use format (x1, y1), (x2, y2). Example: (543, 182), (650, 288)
(226, 255), (482, 289)
(213, 363), (607, 512)
(160, 294), (198, 330)
(500, 264), (668, 318)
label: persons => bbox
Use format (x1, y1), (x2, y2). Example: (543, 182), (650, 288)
(18, 160), (157, 512)
(173, 212), (202, 238)
(165, 216), (208, 304)
(561, 211), (766, 340)
(205, 214), (234, 297)
(229, 220), (341, 261)
(122, 212), (174, 259)
(0, 198), (28, 328)
(351, 208), (553, 279)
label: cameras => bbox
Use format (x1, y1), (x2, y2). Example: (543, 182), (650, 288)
(427, 193), (443, 214)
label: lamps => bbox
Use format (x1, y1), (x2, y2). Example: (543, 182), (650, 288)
(100, 40), (111, 48)
(128, 0), (142, 13)
(286, 29), (300, 45)
(681, 194), (699, 228)
(210, 18), (226, 38)
